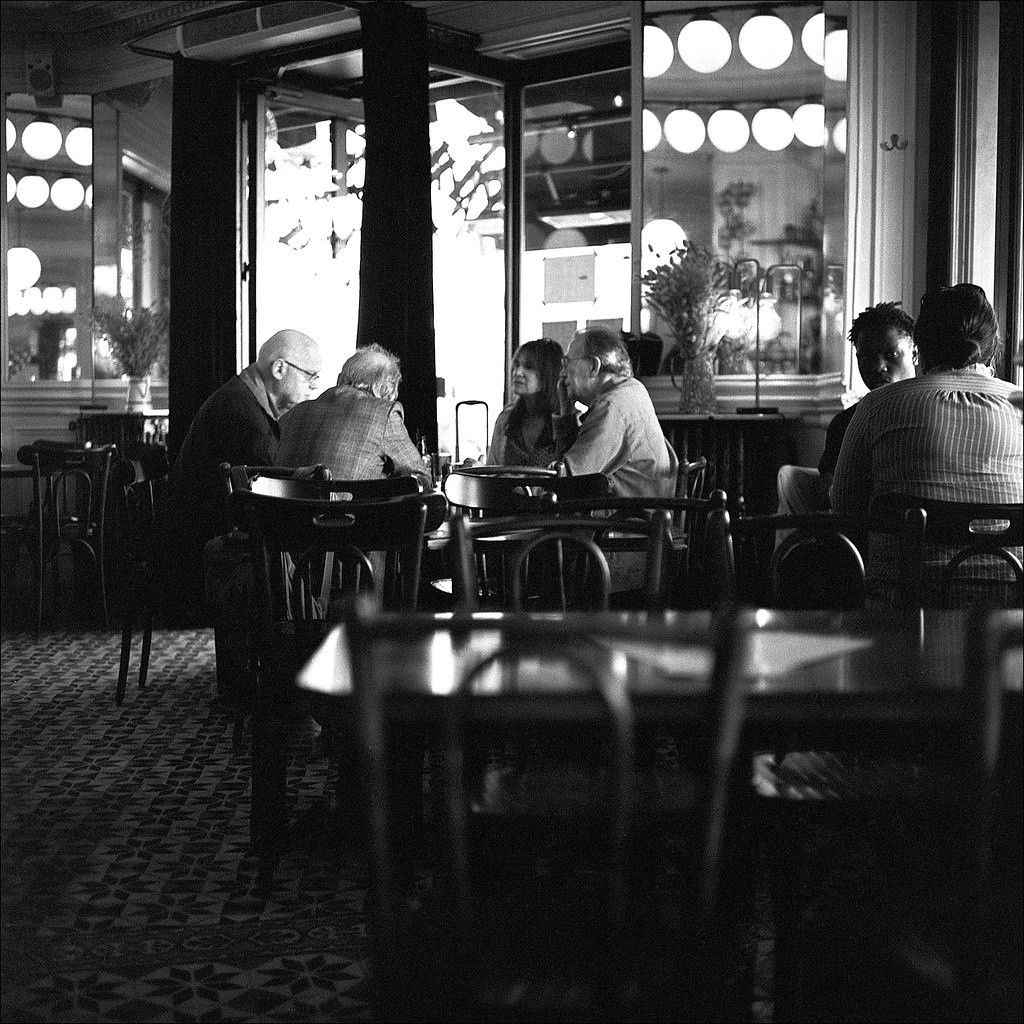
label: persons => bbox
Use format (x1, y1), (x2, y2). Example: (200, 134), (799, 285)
(164, 329), (324, 571)
(275, 344), (430, 609)
(548, 325), (681, 592)
(485, 337), (563, 470)
(829, 283), (1024, 616)
(818, 300), (922, 512)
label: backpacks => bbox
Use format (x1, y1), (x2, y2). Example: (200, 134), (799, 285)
(204, 464), (337, 620)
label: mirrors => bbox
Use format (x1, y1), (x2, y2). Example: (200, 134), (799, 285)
(3, 90), (97, 381)
(634, 3), (849, 384)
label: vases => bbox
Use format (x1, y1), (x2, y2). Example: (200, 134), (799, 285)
(680, 354), (717, 413)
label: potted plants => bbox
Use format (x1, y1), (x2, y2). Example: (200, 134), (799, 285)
(86, 293), (173, 415)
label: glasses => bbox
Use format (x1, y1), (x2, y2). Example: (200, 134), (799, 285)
(271, 357), (319, 381)
(920, 283), (986, 308)
(561, 356), (591, 369)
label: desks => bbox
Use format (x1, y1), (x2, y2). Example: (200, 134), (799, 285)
(654, 412), (788, 518)
(297, 604), (1023, 728)
(0, 461), (87, 479)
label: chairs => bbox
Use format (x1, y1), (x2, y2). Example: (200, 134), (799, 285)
(449, 508), (708, 819)
(774, 608), (1024, 1024)
(540, 482), (724, 608)
(226, 464), (330, 505)
(443, 471), (610, 606)
(661, 455), (708, 597)
(708, 489), (955, 805)
(335, 600), (749, 1024)
(240, 469), (416, 620)
(864, 492), (1024, 610)
(223, 489), (426, 878)
(0, 438), (113, 644)
(106, 445), (227, 701)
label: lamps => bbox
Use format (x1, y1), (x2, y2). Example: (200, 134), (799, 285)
(5, 108), (93, 212)
(638, 3), (852, 159)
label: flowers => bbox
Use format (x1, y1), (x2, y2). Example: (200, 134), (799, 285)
(638, 237), (732, 361)
